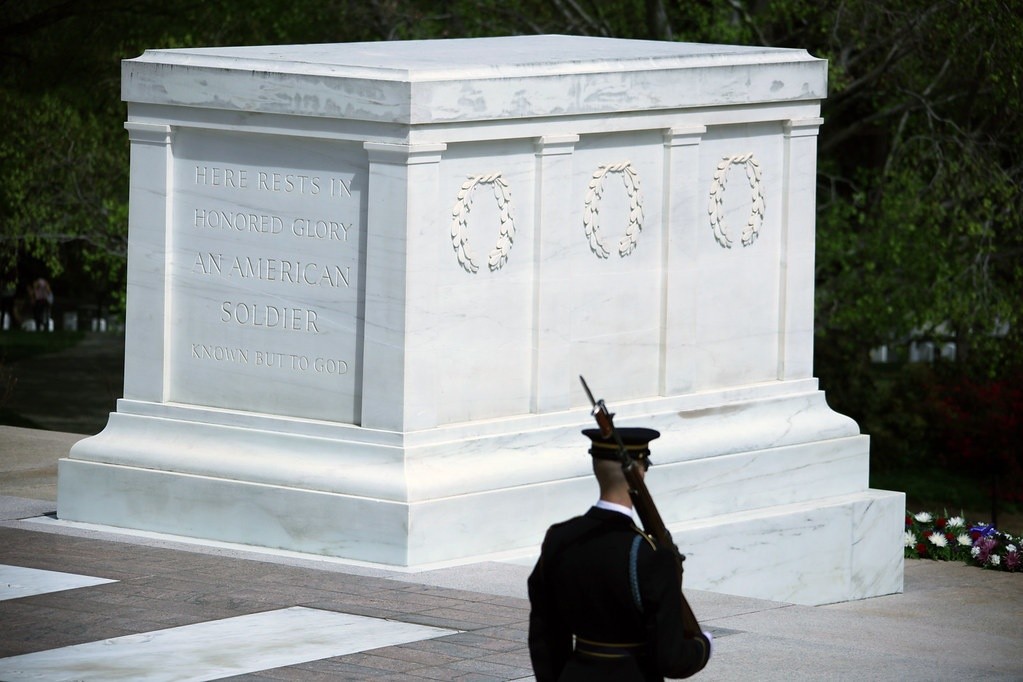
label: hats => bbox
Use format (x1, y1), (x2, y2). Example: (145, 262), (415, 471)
(581, 428), (660, 466)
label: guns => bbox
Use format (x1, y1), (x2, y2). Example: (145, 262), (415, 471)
(579, 375), (701, 634)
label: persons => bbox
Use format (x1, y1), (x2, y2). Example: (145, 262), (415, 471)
(525, 428), (710, 682)
(32, 277), (53, 332)
